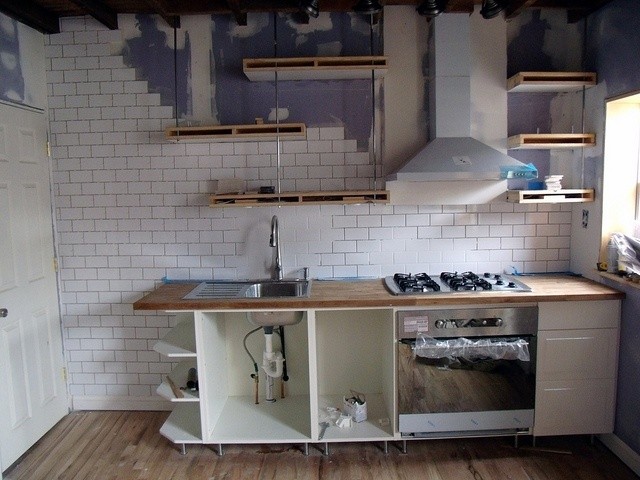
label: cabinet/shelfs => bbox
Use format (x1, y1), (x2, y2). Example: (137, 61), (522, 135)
(152, 310), (202, 455)
(165, 12), (390, 209)
(196, 306), (396, 458)
(533, 300), (621, 446)
(506, 72), (597, 203)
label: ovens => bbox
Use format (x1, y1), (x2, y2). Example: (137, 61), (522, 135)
(393, 305), (538, 437)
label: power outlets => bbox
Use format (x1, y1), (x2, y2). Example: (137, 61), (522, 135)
(582, 210), (589, 221)
(582, 221), (589, 229)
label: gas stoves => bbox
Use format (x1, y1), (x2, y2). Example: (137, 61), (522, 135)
(382, 271), (531, 296)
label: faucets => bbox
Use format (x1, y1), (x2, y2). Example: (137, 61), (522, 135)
(270, 216), (282, 282)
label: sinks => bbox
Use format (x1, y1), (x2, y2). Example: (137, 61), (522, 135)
(241, 281), (311, 299)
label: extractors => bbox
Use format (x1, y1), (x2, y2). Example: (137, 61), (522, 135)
(386, 135), (539, 182)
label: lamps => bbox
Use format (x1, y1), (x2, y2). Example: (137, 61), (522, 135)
(479, 1), (506, 20)
(358, 0), (383, 16)
(302, 0), (320, 18)
(414, 0), (443, 23)
